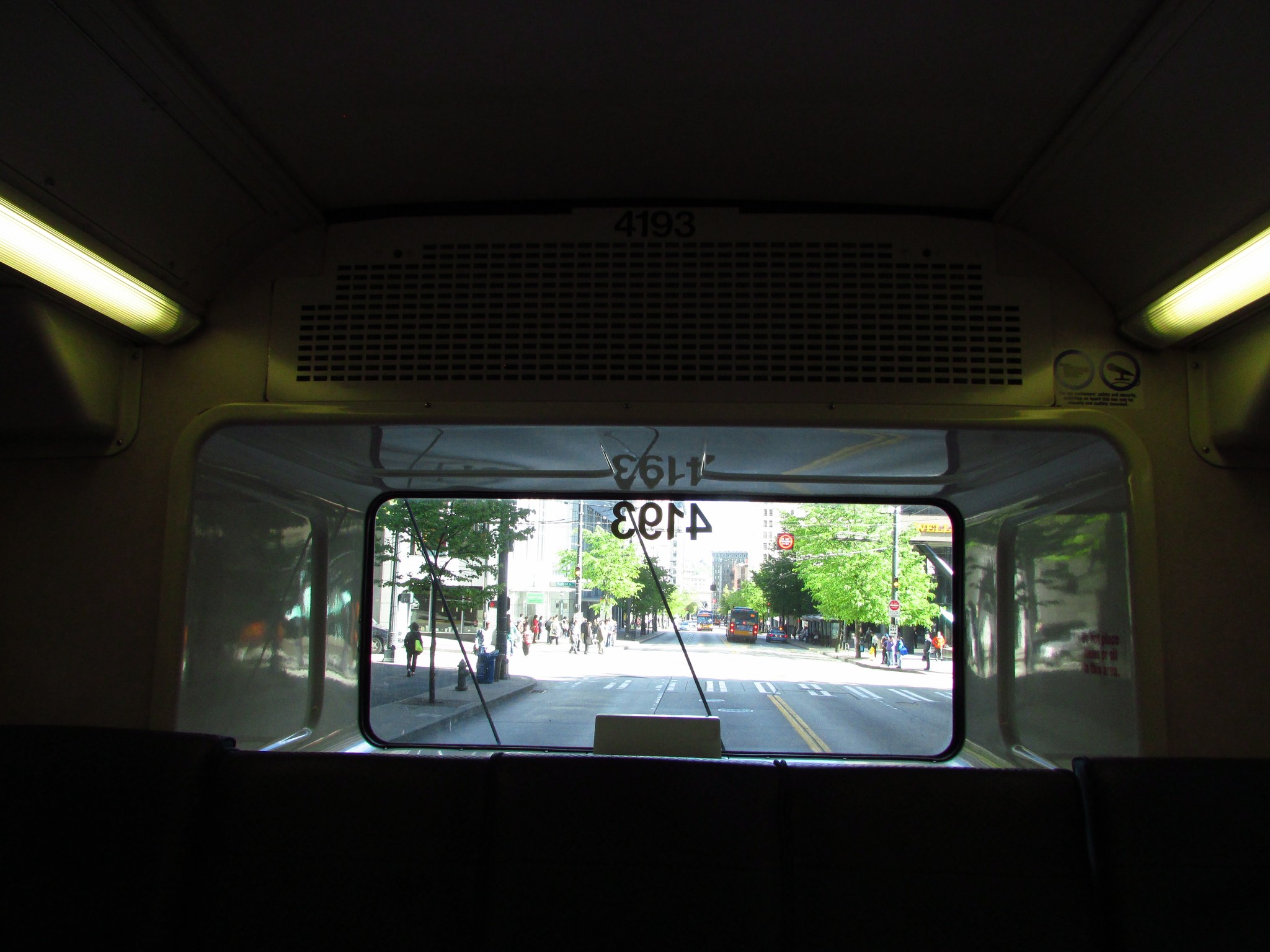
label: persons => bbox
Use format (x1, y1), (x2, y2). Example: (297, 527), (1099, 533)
(793, 625), (819, 644)
(864, 626), (945, 670)
(628, 616), (644, 641)
(475, 612), (617, 671)
(403, 622), (424, 677)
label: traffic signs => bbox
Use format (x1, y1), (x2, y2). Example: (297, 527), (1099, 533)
(889, 625), (898, 636)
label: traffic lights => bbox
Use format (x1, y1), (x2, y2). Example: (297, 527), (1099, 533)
(488, 602), (498, 608)
(575, 566), (580, 580)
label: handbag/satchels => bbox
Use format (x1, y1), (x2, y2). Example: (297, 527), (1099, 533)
(526, 639), (533, 644)
(415, 639), (423, 652)
(552, 629), (558, 635)
(531, 625), (536, 630)
(474, 646), (486, 656)
(922, 655), (927, 661)
(899, 646), (908, 656)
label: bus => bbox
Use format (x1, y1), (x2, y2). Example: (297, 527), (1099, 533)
(725, 606), (759, 644)
(697, 613), (713, 631)
(713, 615), (720, 625)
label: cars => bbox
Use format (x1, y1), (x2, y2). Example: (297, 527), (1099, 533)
(766, 628), (787, 642)
(684, 620), (695, 627)
(691, 620), (698, 626)
(371, 618), (389, 655)
(678, 622), (689, 631)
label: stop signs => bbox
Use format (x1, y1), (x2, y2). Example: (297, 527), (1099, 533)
(889, 600), (900, 617)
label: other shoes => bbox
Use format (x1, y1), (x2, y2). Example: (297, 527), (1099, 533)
(412, 673), (416, 676)
(407, 670), (411, 677)
